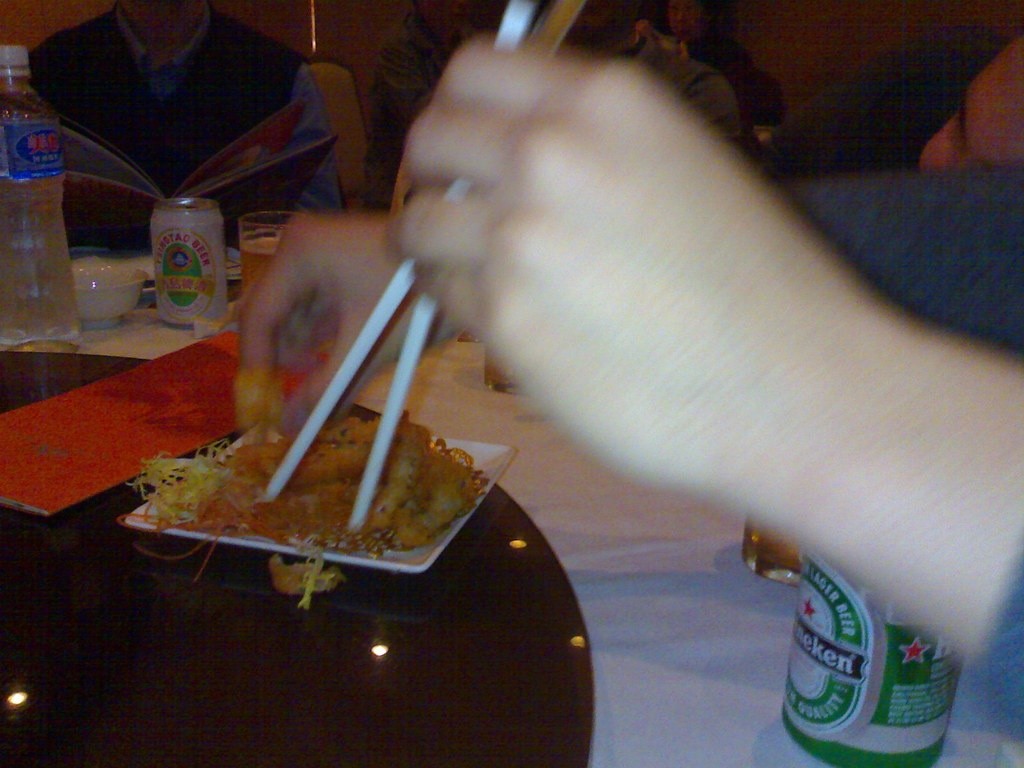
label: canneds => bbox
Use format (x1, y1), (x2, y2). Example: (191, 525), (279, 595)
(149, 197), (229, 331)
(783, 546), (964, 768)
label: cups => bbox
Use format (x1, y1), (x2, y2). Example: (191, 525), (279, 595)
(742, 507), (804, 588)
(238, 212), (341, 352)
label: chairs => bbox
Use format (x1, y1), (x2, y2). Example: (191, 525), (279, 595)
(307, 58), (369, 204)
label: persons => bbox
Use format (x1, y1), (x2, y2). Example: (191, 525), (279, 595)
(240, 38), (1024, 735)
(568, 0), (742, 145)
(30, 0), (342, 215)
(657, 0), (753, 128)
(376, 0), (473, 136)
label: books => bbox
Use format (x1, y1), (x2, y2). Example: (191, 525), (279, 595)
(59, 100), (339, 229)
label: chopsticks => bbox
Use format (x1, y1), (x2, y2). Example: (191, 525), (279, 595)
(252, 0), (584, 539)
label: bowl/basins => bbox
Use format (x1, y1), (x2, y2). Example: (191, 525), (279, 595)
(65, 259), (150, 329)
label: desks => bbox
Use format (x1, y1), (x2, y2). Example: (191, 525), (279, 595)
(1, 251), (1024, 767)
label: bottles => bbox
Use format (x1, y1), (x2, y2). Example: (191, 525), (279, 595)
(0, 44), (79, 344)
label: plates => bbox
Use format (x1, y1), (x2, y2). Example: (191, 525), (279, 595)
(124, 438), (517, 572)
